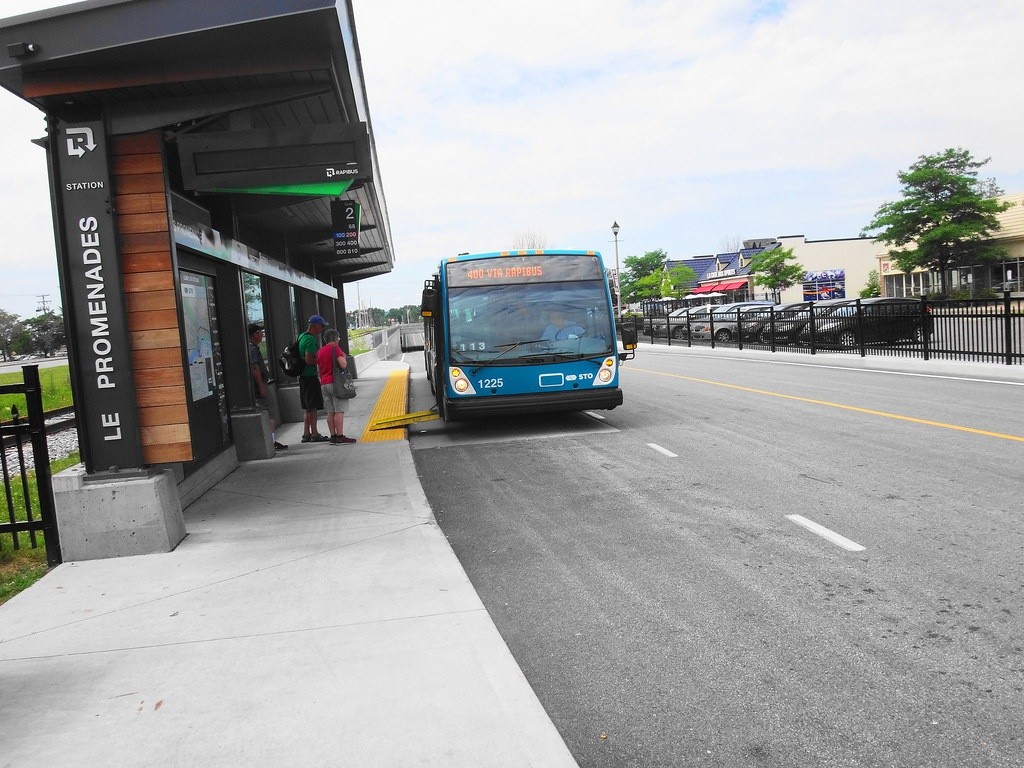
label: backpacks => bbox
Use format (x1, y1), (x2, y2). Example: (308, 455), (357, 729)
(279, 332), (318, 377)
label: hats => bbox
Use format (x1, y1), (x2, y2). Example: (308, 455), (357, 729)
(308, 315), (329, 325)
(248, 323), (265, 336)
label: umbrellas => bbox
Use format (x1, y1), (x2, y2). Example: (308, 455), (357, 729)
(656, 292), (727, 307)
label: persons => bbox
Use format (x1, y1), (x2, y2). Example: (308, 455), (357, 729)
(540, 307), (586, 341)
(296, 314), (331, 443)
(247, 323), (288, 450)
(315, 329), (357, 445)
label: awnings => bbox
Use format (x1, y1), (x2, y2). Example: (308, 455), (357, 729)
(693, 281), (748, 293)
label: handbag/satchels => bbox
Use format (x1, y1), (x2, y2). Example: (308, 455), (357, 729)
(332, 345), (356, 399)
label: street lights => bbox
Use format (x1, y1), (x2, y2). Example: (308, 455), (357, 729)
(611, 221), (621, 323)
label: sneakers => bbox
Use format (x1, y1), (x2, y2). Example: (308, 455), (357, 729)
(311, 433), (330, 442)
(336, 434), (356, 446)
(301, 434), (311, 443)
(273, 441), (288, 450)
(330, 434), (337, 445)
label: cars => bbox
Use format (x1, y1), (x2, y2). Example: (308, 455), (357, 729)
(622, 296), (935, 348)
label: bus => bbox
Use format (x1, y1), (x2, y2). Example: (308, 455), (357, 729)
(421, 250), (638, 421)
